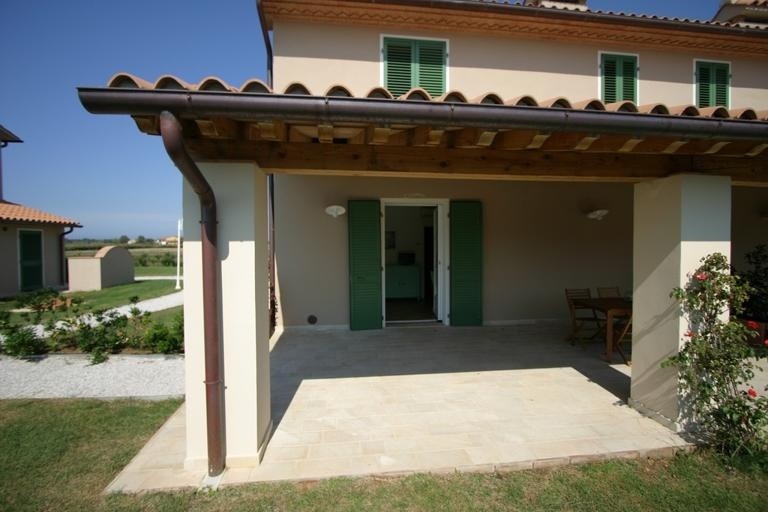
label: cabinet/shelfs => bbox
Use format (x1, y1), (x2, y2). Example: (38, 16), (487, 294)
(386, 263), (421, 303)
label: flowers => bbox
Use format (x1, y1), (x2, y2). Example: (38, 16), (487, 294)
(662, 249), (767, 457)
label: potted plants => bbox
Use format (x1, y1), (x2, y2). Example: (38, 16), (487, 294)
(731, 241), (768, 347)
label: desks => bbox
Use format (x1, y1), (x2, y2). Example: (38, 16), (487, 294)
(572, 297), (633, 363)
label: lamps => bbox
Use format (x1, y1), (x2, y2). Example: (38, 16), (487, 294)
(325, 204), (346, 219)
(585, 208), (609, 221)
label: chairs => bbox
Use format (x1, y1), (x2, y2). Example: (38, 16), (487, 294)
(565, 286), (632, 345)
